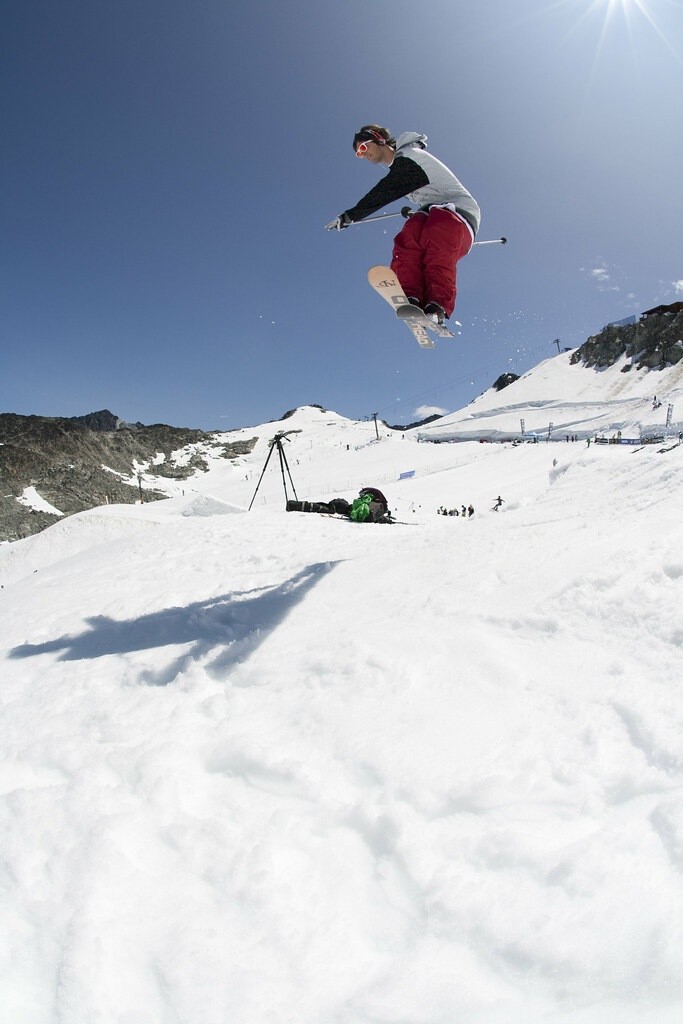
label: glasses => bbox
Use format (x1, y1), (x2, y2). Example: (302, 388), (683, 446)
(356, 138), (376, 159)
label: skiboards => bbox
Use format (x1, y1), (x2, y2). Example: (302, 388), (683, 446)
(366, 264), (454, 349)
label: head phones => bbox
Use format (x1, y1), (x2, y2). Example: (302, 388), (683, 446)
(364, 129), (386, 145)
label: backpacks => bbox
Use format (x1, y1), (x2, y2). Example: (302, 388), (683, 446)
(286, 488), (395, 524)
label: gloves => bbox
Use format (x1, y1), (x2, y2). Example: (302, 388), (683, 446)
(325, 212), (352, 232)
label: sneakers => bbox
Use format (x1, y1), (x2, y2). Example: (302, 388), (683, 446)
(407, 298), (422, 309)
(423, 305), (446, 323)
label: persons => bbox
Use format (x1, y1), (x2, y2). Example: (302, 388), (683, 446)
(326, 126), (480, 321)
(532, 429), (622, 448)
(435, 493), (504, 515)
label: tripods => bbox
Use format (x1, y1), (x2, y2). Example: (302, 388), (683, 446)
(247, 434), (299, 512)
